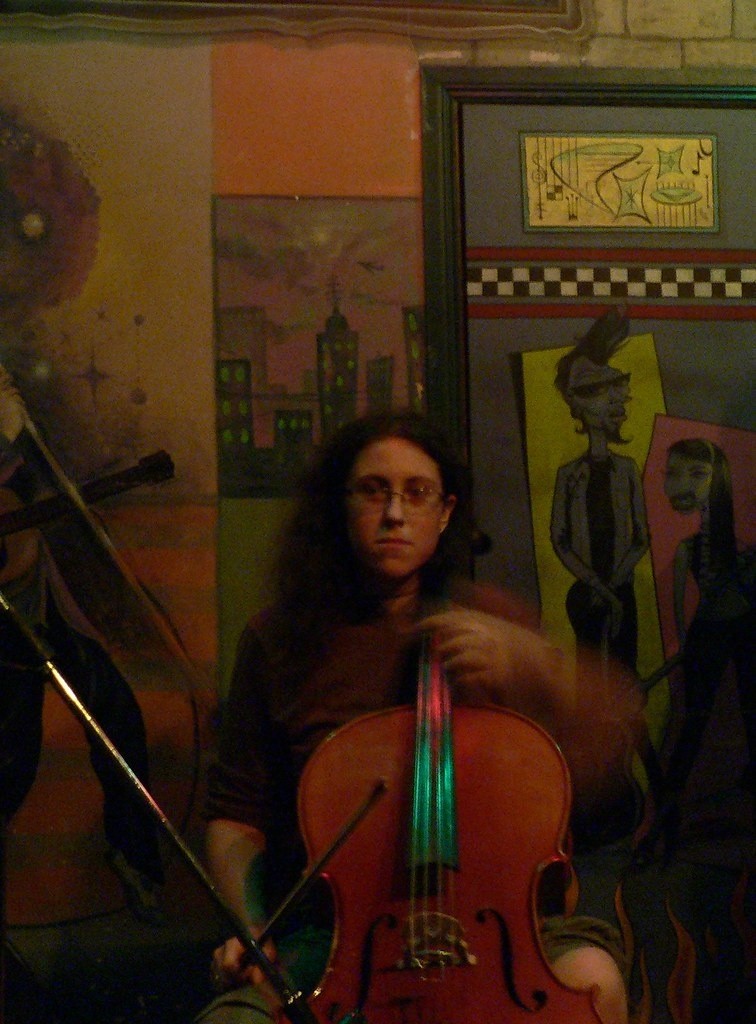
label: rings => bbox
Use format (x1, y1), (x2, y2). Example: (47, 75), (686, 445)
(211, 973), (225, 983)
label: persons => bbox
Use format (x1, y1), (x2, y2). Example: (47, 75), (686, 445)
(190, 406), (648, 1024)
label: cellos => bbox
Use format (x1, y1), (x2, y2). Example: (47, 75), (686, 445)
(263, 462), (608, 1024)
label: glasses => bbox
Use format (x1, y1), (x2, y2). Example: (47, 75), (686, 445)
(346, 483), (444, 507)
